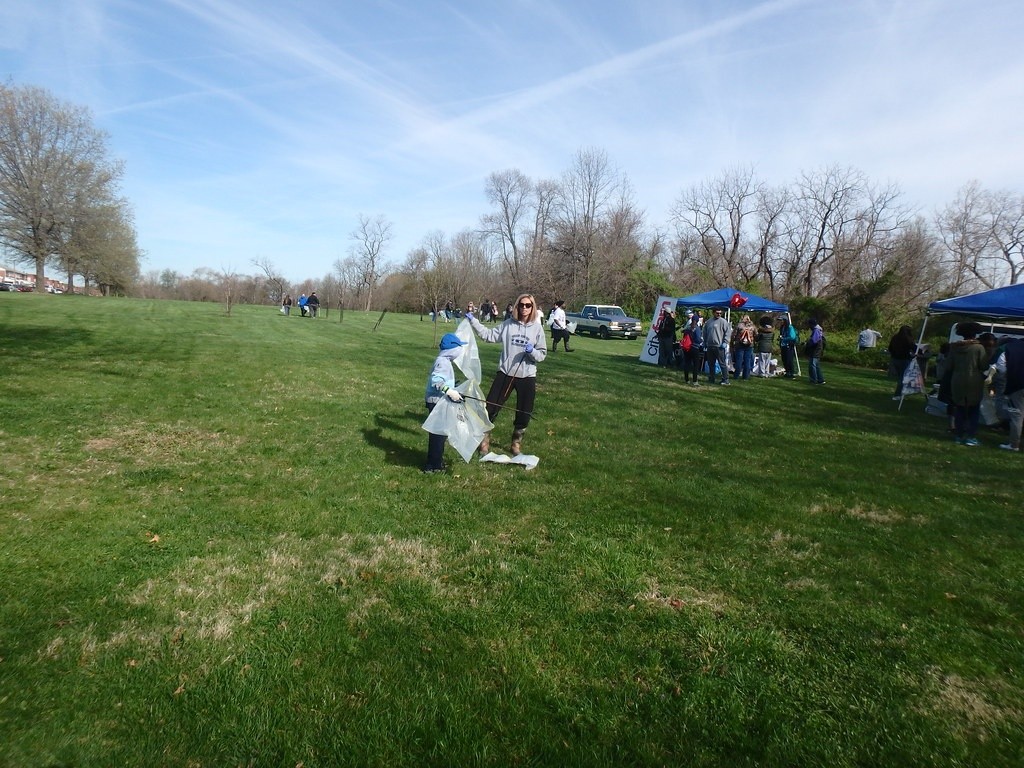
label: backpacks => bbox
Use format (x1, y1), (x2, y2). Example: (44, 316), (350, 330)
(739, 327), (752, 346)
(792, 329), (800, 344)
(821, 336), (826, 350)
(679, 333), (692, 352)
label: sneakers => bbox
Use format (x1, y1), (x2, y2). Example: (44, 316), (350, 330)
(999, 442), (1020, 451)
(957, 436), (966, 442)
(964, 437), (982, 446)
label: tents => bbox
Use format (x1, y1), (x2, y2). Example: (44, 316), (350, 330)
(898, 283), (1024, 412)
(677, 287), (801, 372)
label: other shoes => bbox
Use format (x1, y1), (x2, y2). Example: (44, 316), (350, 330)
(510, 443), (521, 455)
(480, 433), (490, 453)
(891, 396), (902, 400)
(693, 381), (699, 386)
(721, 381), (731, 386)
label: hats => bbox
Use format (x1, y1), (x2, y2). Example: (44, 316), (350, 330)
(439, 334), (468, 350)
(662, 306), (671, 313)
(556, 300), (565, 307)
(692, 315), (699, 320)
(777, 315), (789, 321)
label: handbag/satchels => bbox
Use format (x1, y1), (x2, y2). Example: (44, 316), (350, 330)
(902, 358), (925, 395)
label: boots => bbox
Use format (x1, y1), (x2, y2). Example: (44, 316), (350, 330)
(553, 342), (557, 352)
(564, 341), (575, 352)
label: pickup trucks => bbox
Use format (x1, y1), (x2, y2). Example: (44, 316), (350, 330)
(565, 305), (643, 341)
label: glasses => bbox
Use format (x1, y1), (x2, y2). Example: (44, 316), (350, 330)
(518, 302), (532, 308)
(715, 312), (722, 314)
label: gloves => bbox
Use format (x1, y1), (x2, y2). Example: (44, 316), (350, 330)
(722, 342), (727, 348)
(526, 343), (534, 354)
(446, 388), (463, 401)
(466, 312), (474, 320)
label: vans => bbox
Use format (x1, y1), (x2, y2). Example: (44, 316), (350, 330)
(948, 322), (1024, 343)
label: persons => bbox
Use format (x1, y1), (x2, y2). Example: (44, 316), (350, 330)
(455, 308), (462, 324)
(657, 307), (796, 386)
(546, 300), (575, 352)
(445, 300), (453, 323)
(467, 302), (474, 313)
(937, 324), (1024, 451)
(425, 334), (468, 472)
(505, 301), (513, 320)
(283, 295), (292, 315)
(537, 307), (544, 326)
(298, 292), (321, 318)
(481, 299), (499, 324)
(806, 318), (826, 384)
(465, 294), (547, 455)
(888, 325), (922, 400)
(431, 301), (436, 322)
(858, 324), (881, 350)
